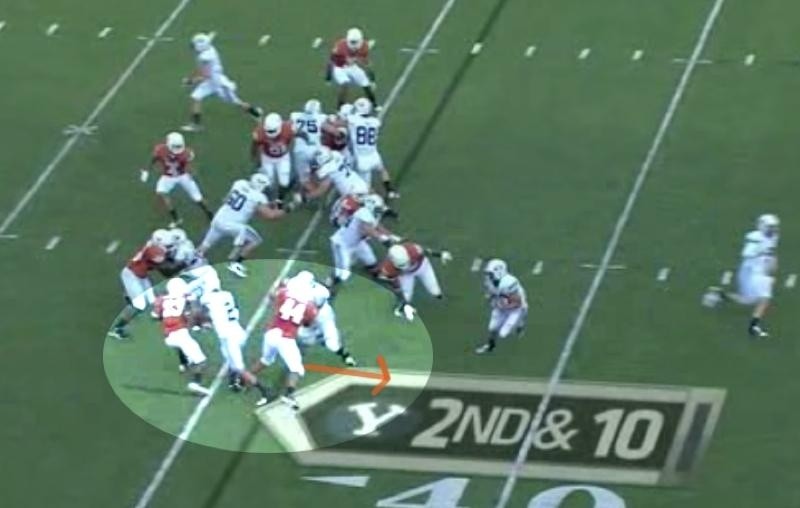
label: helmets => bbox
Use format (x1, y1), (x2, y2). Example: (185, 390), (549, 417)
(151, 229), (189, 247)
(758, 213), (778, 232)
(165, 276), (186, 298)
(193, 34), (210, 52)
(247, 97), (387, 214)
(483, 259), (506, 280)
(167, 132), (184, 154)
(280, 270), (315, 296)
(347, 28), (364, 51)
(388, 244), (409, 270)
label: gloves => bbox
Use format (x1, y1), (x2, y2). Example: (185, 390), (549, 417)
(441, 250), (451, 265)
(404, 303), (417, 320)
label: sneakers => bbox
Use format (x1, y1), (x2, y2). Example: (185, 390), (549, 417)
(280, 394), (300, 409)
(107, 325), (129, 339)
(475, 344), (496, 354)
(340, 351), (358, 371)
(749, 324), (768, 337)
(181, 123), (198, 132)
(225, 257), (249, 278)
(188, 382), (211, 396)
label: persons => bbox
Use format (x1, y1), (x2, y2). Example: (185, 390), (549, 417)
(231, 271), (356, 410)
(379, 240), (454, 315)
(181, 32), (263, 131)
(248, 99), (400, 289)
(109, 228), (271, 406)
(702, 213), (781, 338)
(140, 132), (213, 228)
(323, 28), (381, 112)
(198, 173), (293, 277)
(474, 257), (529, 354)
